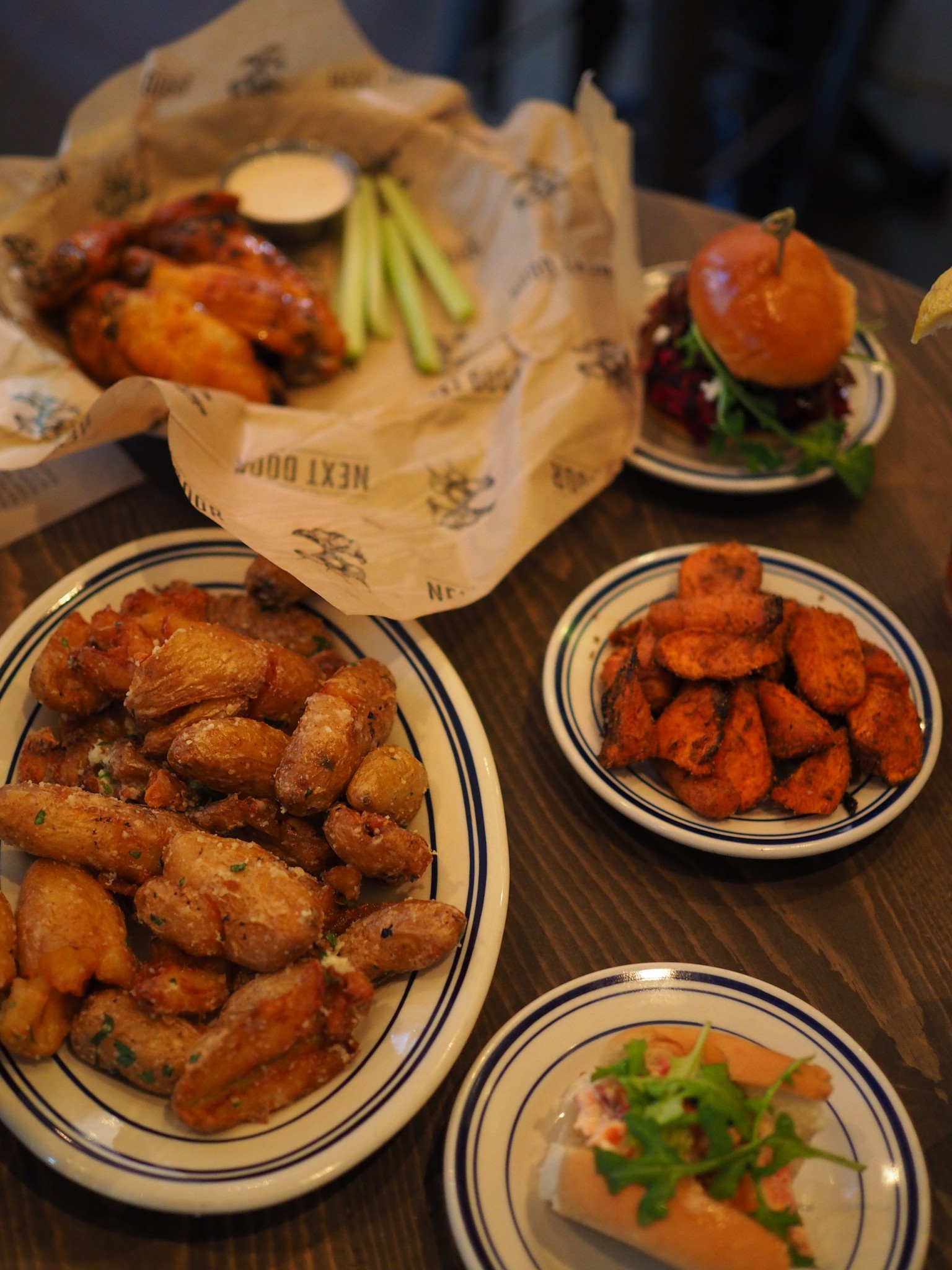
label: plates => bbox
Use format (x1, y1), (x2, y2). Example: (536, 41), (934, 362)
(0, 529), (509, 1217)
(44, 80), (543, 464)
(542, 542), (945, 859)
(442, 963), (931, 1270)
(625, 260), (895, 490)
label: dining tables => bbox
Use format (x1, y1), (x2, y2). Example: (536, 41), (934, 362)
(0, 176), (952, 1270)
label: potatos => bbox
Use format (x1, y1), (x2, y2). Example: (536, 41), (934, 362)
(595, 538), (924, 820)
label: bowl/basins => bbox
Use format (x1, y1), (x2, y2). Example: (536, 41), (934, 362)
(220, 137), (361, 245)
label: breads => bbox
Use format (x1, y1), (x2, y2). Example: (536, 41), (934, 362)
(687, 224), (859, 391)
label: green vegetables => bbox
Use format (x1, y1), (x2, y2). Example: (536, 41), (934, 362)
(679, 323), (903, 499)
(589, 1018), (868, 1269)
